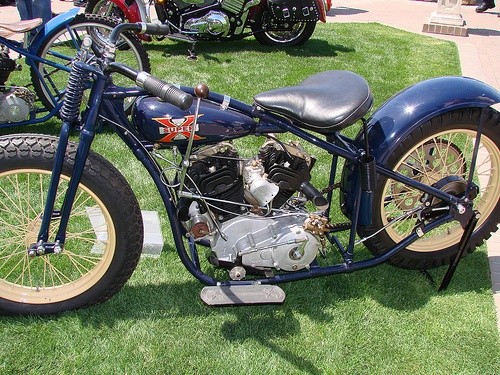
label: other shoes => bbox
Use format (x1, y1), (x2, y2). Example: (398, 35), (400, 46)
(476, 1), (495, 12)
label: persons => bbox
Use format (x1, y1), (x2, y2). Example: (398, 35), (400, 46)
(17, 0), (51, 48)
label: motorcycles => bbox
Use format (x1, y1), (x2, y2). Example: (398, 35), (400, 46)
(83, 0), (332, 61)
(0, 22), (500, 317)
(1, 11), (150, 128)
